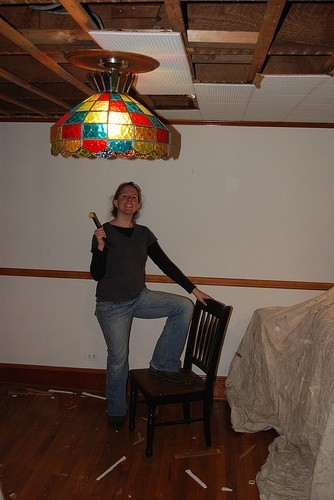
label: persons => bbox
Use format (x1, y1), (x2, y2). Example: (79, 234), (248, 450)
(90, 182), (213, 432)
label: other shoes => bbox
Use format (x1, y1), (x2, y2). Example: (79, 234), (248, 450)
(150, 366), (184, 382)
(107, 415), (125, 427)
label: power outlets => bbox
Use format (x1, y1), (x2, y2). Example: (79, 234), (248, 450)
(86, 352), (96, 360)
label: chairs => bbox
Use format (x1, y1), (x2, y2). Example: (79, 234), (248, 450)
(128, 297), (234, 457)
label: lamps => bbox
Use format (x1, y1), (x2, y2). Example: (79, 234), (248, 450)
(48, 49), (173, 161)
